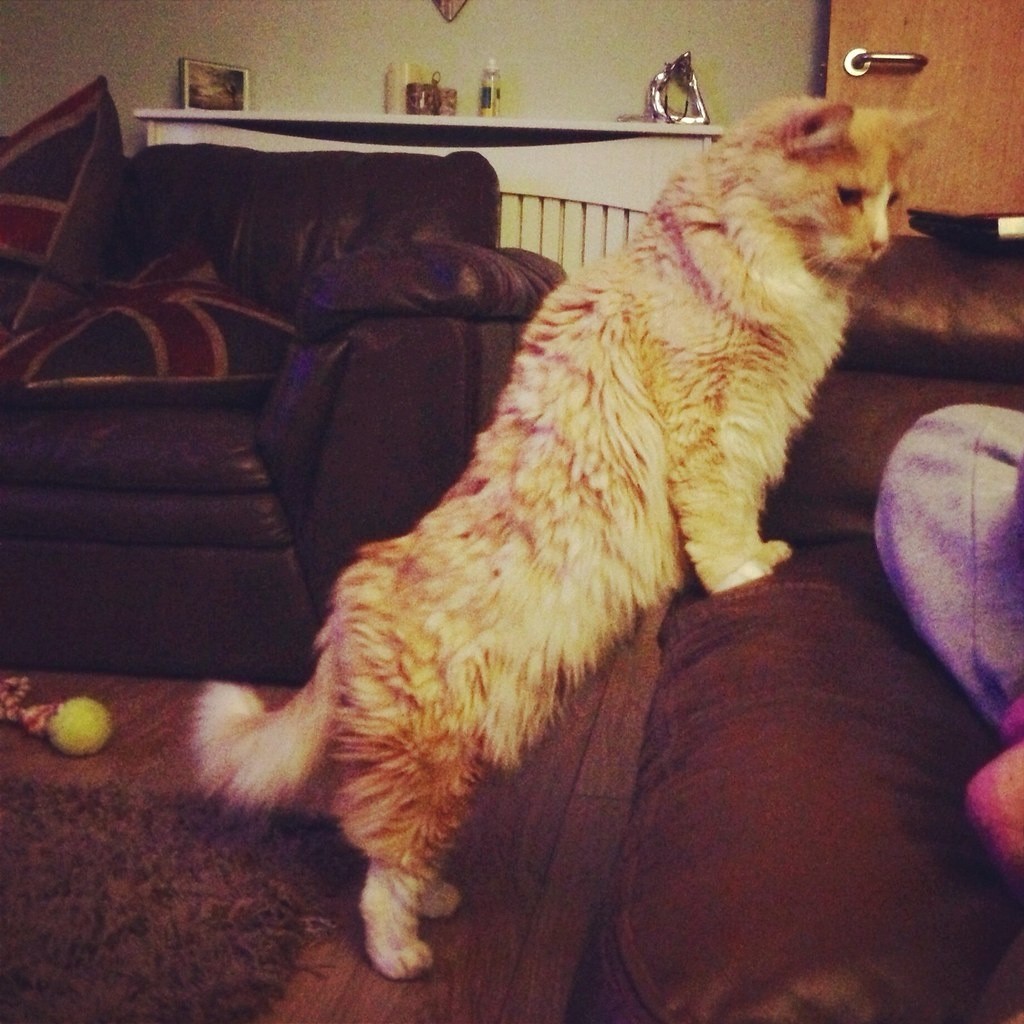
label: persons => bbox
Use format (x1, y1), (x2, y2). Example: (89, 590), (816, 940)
(873, 403), (1024, 870)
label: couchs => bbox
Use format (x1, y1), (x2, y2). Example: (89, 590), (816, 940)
(564, 232), (1024, 1024)
(0, 142), (568, 688)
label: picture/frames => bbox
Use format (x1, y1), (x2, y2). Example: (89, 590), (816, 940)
(178, 58), (250, 112)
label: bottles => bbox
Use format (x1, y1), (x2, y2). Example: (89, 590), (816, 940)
(384, 61), (422, 115)
(480, 58), (502, 117)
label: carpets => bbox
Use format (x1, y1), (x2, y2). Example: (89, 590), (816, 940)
(0, 777), (354, 1024)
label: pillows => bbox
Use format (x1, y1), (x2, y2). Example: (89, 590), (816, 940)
(0, 234), (297, 403)
(0, 76), (124, 331)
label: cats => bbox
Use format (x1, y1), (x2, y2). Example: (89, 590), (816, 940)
(190, 96), (934, 980)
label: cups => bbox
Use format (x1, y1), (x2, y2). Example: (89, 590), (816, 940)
(435, 88), (457, 116)
(406, 83), (440, 116)
